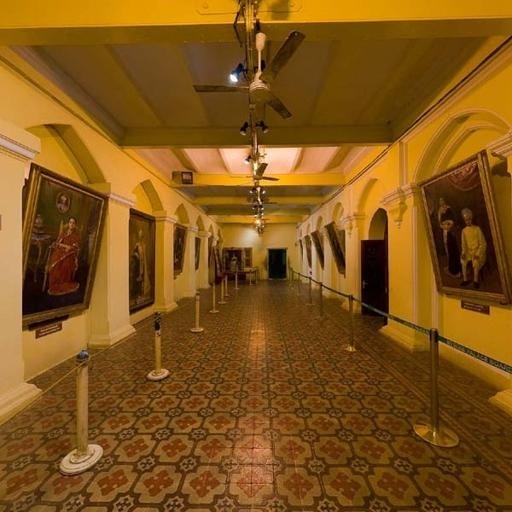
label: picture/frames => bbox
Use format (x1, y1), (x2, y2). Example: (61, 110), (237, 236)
(129, 206), (155, 315)
(416, 149), (512, 310)
(173, 223), (188, 277)
(22, 161), (108, 328)
(222, 246), (253, 274)
(310, 220), (345, 275)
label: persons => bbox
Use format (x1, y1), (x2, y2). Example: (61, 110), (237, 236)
(44, 216), (82, 297)
(437, 195), (462, 278)
(459, 206), (488, 290)
(174, 232), (182, 270)
(132, 229), (146, 299)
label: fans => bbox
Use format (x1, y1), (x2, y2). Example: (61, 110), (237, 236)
(192, 31), (306, 120)
(233, 161), (280, 222)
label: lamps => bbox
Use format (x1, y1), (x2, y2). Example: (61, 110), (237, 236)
(240, 121), (269, 137)
(226, 63), (245, 83)
